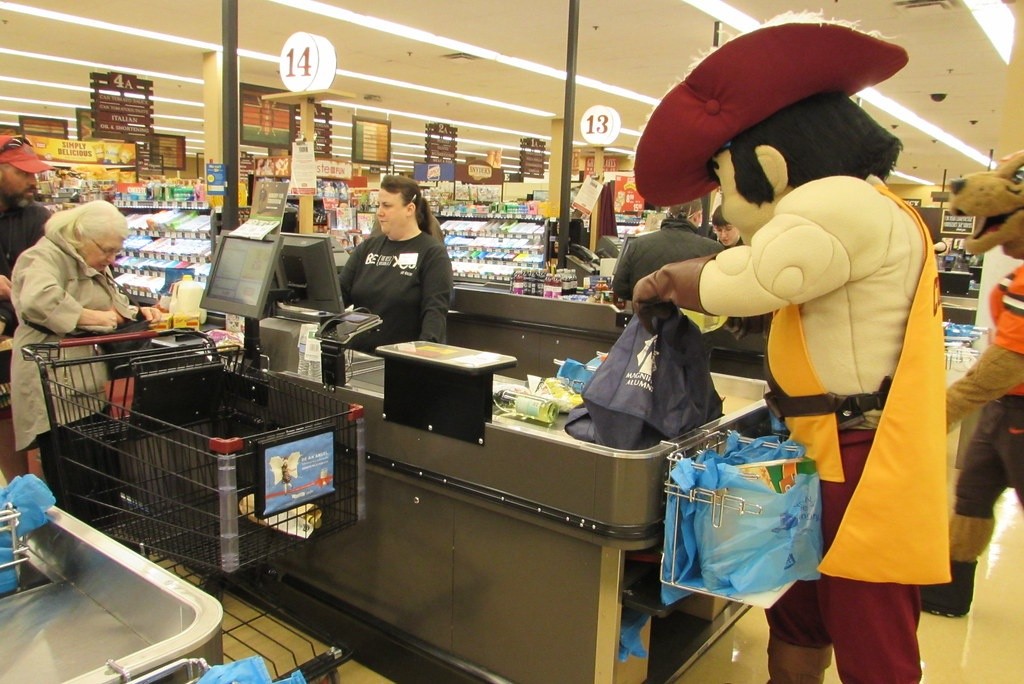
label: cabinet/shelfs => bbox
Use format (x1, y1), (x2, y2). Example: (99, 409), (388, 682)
(435, 199), (591, 287)
(614, 210), (668, 240)
(58, 192), (211, 306)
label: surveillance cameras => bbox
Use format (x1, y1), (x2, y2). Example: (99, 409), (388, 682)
(931, 93), (946, 102)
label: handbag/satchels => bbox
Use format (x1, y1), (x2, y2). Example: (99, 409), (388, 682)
(96, 320), (160, 380)
(565, 298), (724, 448)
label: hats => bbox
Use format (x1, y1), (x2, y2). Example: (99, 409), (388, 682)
(0, 134), (58, 173)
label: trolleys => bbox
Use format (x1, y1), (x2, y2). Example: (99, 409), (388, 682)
(20, 325), (367, 684)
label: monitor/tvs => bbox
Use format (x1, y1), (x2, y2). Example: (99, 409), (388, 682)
(199, 232), (284, 319)
(273, 233), (345, 314)
(613, 234), (643, 275)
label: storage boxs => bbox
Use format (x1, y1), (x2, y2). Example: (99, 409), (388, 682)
(337, 209), (377, 237)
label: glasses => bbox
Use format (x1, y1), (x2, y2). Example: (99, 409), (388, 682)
(89, 238), (124, 257)
(0, 137), (33, 154)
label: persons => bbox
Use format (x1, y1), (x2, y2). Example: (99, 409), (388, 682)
(10, 200), (161, 531)
(611, 201), (727, 358)
(712, 204), (745, 249)
(0, 133), (52, 481)
(631, 12), (952, 684)
(339, 175), (454, 356)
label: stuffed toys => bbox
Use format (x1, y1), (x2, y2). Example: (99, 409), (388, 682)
(917, 151), (1024, 616)
